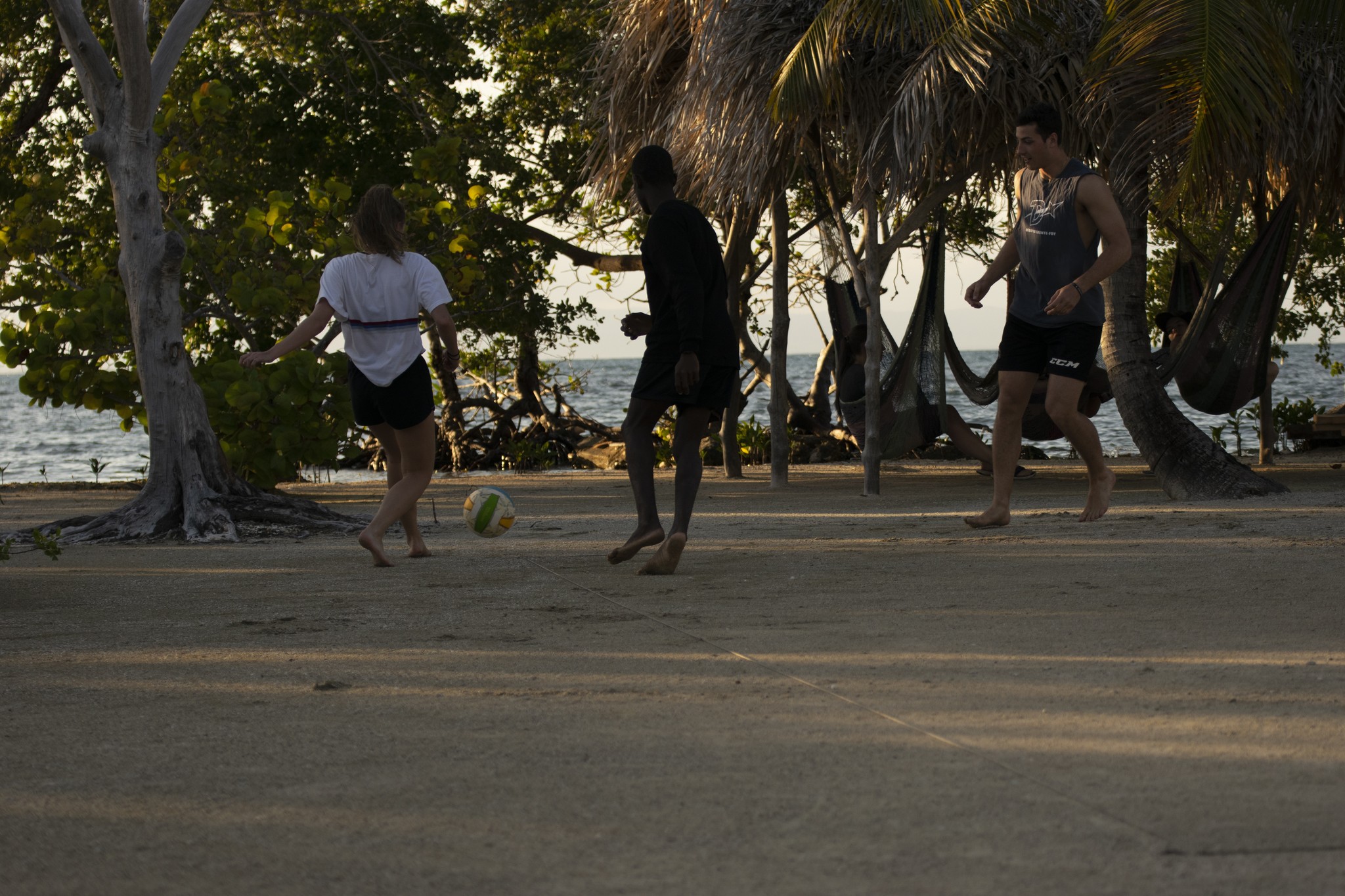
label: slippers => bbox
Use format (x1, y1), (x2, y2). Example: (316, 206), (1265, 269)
(991, 466), (1036, 480)
(976, 469), (993, 476)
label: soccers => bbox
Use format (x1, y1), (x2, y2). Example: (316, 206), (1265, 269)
(463, 486), (516, 538)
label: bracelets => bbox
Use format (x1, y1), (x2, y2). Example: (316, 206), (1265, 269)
(446, 350), (460, 360)
(1070, 282), (1083, 298)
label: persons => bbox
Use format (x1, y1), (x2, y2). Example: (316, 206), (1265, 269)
(606, 145), (740, 575)
(840, 325), (1037, 480)
(964, 103), (1132, 527)
(1155, 310), (1279, 415)
(239, 184), (460, 566)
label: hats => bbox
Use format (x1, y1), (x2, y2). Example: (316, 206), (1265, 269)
(1155, 311), (1193, 337)
